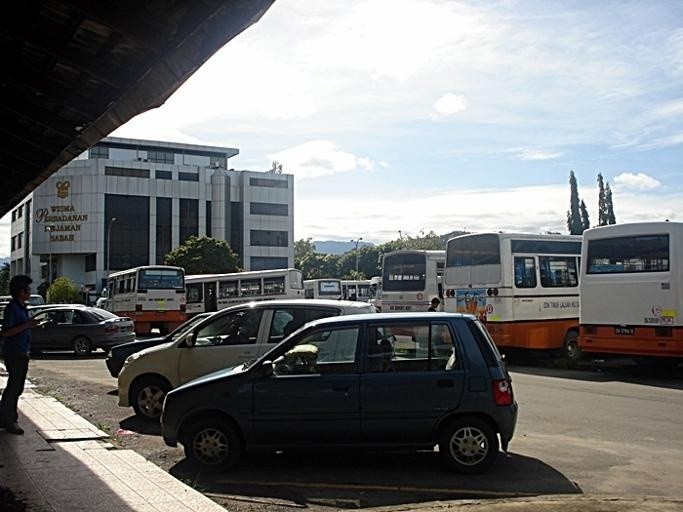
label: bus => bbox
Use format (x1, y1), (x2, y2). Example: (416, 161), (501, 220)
(95, 263), (385, 336)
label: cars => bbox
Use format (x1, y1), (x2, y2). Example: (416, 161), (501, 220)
(0, 303), (135, 358)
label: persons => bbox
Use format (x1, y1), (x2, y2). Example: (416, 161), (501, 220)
(428, 297), (440, 311)
(0, 274), (43, 435)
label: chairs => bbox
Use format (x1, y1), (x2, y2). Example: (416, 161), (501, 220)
(369, 337), (458, 372)
(220, 320), (307, 343)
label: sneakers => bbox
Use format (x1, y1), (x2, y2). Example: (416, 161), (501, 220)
(5, 420), (24, 435)
